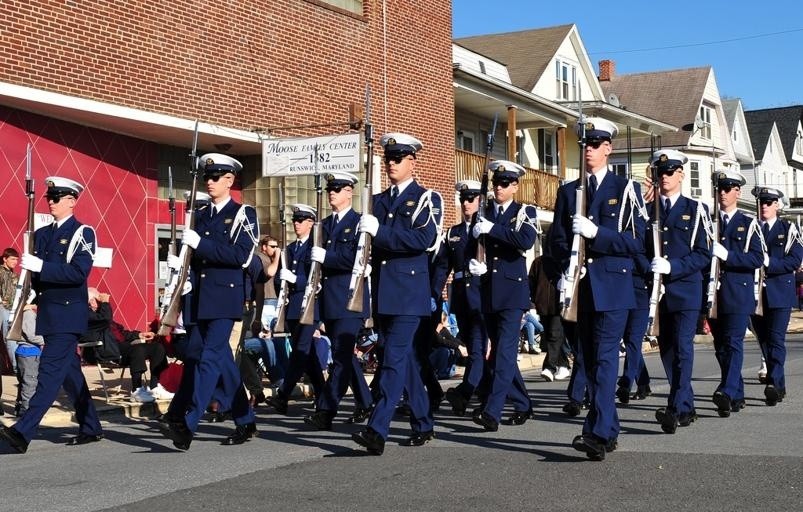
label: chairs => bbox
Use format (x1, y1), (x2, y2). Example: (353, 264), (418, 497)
(78, 339), (150, 405)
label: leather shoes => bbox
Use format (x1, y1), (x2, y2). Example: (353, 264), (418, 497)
(733, 396), (748, 411)
(563, 404), (581, 416)
(399, 426), (436, 448)
(763, 384), (778, 407)
(617, 389), (631, 403)
(209, 410), (234, 422)
(351, 429), (384, 454)
(0, 423), (28, 454)
(265, 396), (287, 413)
(227, 422), (259, 445)
(571, 435), (607, 462)
(446, 387), (466, 417)
(678, 411), (700, 429)
(634, 389), (653, 400)
(303, 412), (333, 430)
(348, 404), (375, 423)
(69, 431), (106, 445)
(654, 408), (677, 432)
(712, 390), (732, 418)
(511, 410), (537, 424)
(249, 392), (265, 406)
(606, 436), (620, 452)
(159, 418), (191, 450)
(471, 412), (498, 431)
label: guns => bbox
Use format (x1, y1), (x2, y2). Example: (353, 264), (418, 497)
(646, 132), (661, 338)
(298, 142), (324, 327)
(345, 82), (375, 314)
(165, 164), (186, 335)
(560, 76), (587, 323)
(4, 141), (38, 344)
(157, 117), (200, 338)
(708, 141), (719, 319)
(273, 182), (287, 334)
(753, 162), (763, 317)
(476, 113), (501, 264)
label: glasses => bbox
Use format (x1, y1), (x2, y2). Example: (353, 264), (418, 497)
(459, 196), (481, 204)
(658, 168), (682, 177)
(326, 187), (347, 194)
(202, 174), (222, 182)
(267, 245), (279, 249)
(48, 194), (72, 204)
(760, 198), (777, 206)
(292, 217), (311, 224)
(384, 156), (413, 167)
(718, 185), (739, 194)
(492, 180), (514, 189)
(585, 139), (607, 149)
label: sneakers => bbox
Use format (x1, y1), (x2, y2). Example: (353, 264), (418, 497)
(555, 367), (571, 380)
(540, 368), (554, 382)
(146, 383), (175, 400)
(130, 388), (154, 404)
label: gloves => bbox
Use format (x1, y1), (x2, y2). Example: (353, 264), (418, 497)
(311, 246), (326, 264)
(359, 213), (380, 237)
(472, 216), (495, 239)
(181, 228), (202, 249)
(280, 268), (297, 283)
(651, 257), (672, 275)
(659, 284), (666, 302)
(580, 266), (587, 279)
(763, 252), (770, 267)
(469, 258), (488, 276)
(359, 263), (372, 277)
(706, 281), (721, 293)
(305, 283), (322, 297)
(181, 280), (193, 295)
(572, 213), (598, 239)
(19, 253), (43, 272)
(710, 242), (728, 261)
(168, 254), (180, 270)
(429, 298), (437, 311)
(556, 279), (562, 292)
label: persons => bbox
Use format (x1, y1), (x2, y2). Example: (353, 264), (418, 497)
(244, 322), (294, 400)
(704, 169), (768, 418)
(0, 246), (20, 375)
(205, 253), (266, 415)
(0, 175), (100, 454)
(641, 150), (716, 434)
(464, 159), (543, 431)
(13, 292), (45, 417)
(529, 255), (572, 383)
(310, 329), (330, 379)
(160, 191), (232, 422)
(265, 202), (324, 413)
(615, 256), (658, 404)
(304, 171), (374, 432)
(552, 116), (652, 459)
(432, 310), (469, 379)
(430, 179), (488, 417)
(521, 307), (545, 354)
(157, 152), (261, 451)
(352, 131), (445, 456)
(753, 185), (803, 408)
(80, 286), (176, 404)
(253, 236), (285, 309)
(441, 288), (460, 338)
(747, 314), (769, 383)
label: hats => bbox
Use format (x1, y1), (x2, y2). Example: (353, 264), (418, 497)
(575, 116), (619, 137)
(379, 132), (424, 152)
(453, 180), (481, 195)
(324, 170), (360, 184)
(183, 190), (211, 205)
(648, 150), (688, 167)
(44, 175), (85, 192)
(288, 203), (318, 215)
(713, 168), (747, 184)
(487, 159), (527, 177)
(486, 189), (496, 207)
(198, 152), (243, 171)
(751, 186), (786, 198)
